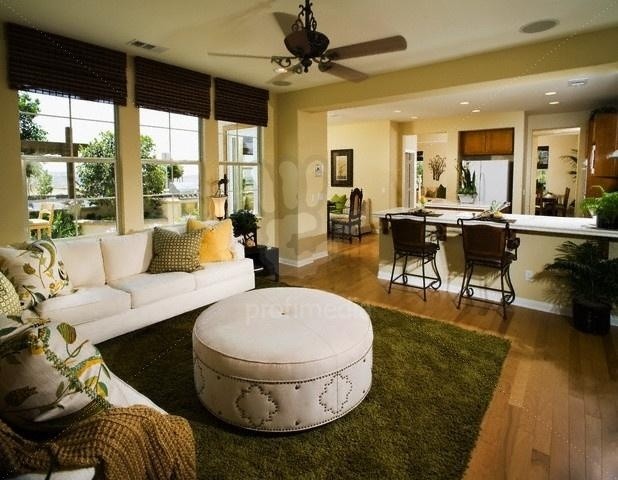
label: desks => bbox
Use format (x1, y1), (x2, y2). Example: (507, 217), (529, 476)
(373, 206), (617, 325)
(328, 200), (336, 236)
(192, 286), (375, 434)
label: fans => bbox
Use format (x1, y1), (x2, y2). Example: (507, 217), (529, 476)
(207, 0), (407, 86)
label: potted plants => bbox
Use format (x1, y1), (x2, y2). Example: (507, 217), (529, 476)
(454, 158), (478, 204)
(576, 184), (617, 231)
(530, 233), (618, 334)
(230, 209), (259, 245)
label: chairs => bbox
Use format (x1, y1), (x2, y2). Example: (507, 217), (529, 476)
(385, 211), (442, 304)
(331, 186), (364, 244)
(28, 202), (55, 240)
(546, 187), (570, 216)
(535, 189), (543, 215)
(456, 215), (521, 321)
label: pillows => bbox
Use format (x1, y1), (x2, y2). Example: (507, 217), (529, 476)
(0, 319), (112, 423)
(0, 234), (78, 310)
(188, 218), (235, 263)
(0, 270), (23, 319)
(330, 194), (346, 214)
(146, 226), (206, 274)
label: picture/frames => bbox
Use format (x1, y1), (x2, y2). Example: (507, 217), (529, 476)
(330, 149), (353, 187)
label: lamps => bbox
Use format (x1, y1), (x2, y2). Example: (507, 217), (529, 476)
(211, 174), (229, 221)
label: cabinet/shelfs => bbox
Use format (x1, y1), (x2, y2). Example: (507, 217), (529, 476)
(460, 126), (513, 157)
(245, 246), (280, 284)
(584, 109), (617, 201)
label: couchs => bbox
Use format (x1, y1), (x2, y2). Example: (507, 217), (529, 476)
(1, 374), (195, 480)
(328, 194), (371, 237)
(0, 221), (255, 345)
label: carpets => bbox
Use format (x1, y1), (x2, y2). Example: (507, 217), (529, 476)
(92, 281), (512, 479)
